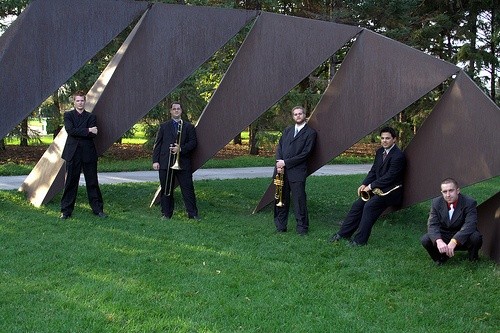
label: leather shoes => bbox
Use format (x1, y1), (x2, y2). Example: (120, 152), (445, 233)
(161, 215), (169, 220)
(189, 215), (201, 221)
(98, 212), (108, 218)
(60, 212), (72, 219)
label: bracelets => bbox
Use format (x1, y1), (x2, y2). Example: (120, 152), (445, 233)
(451, 239), (457, 245)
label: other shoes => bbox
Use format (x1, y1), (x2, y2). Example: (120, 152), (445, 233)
(471, 257), (478, 262)
(349, 240), (357, 245)
(331, 233), (342, 240)
(438, 255), (449, 266)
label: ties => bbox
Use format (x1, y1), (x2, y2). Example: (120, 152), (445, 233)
(448, 204), (455, 221)
(383, 151), (387, 162)
(296, 129), (298, 136)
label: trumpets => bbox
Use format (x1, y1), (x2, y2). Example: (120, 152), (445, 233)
(274, 173), (284, 207)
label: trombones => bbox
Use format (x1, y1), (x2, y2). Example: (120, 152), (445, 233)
(164, 119), (183, 196)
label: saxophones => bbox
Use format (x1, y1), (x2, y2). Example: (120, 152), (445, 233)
(360, 185), (402, 202)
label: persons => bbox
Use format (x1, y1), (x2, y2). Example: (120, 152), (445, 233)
(421, 178), (483, 267)
(152, 101), (202, 222)
(59, 91), (108, 220)
(271, 106), (317, 237)
(332, 126), (408, 247)
